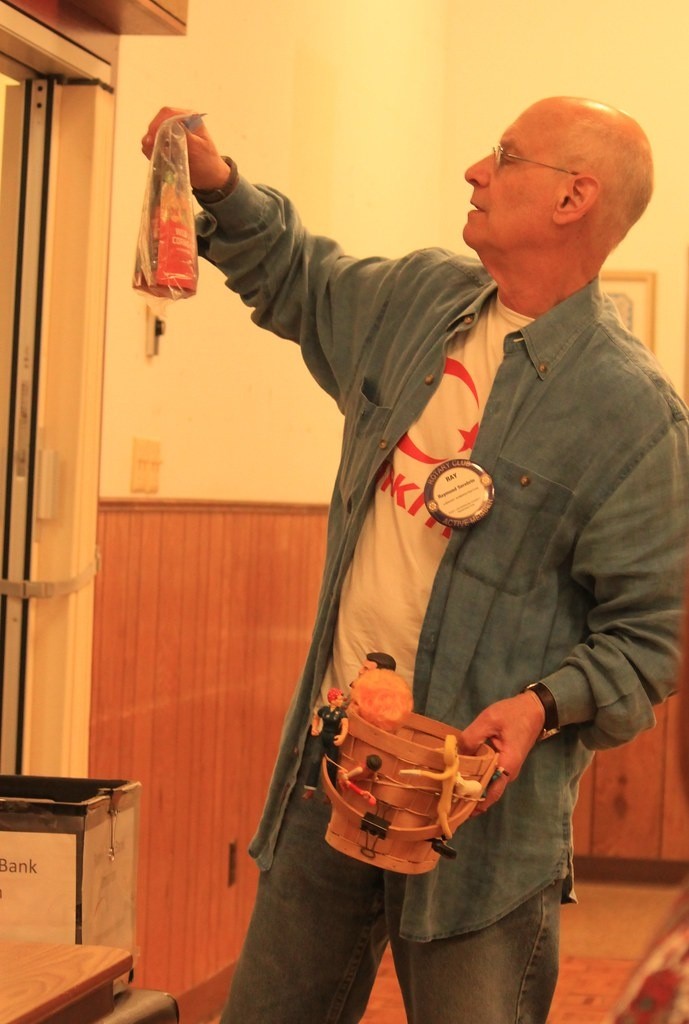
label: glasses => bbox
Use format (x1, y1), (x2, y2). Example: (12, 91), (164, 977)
(493, 145), (579, 179)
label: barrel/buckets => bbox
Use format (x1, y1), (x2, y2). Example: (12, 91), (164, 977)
(322, 706), (502, 876)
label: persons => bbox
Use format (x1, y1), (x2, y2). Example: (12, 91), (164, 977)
(338, 753), (383, 807)
(358, 653), (396, 678)
(140, 97), (689, 1023)
(302, 688), (349, 803)
(399, 735), (459, 840)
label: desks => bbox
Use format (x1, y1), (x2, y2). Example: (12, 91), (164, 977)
(1, 940), (180, 1024)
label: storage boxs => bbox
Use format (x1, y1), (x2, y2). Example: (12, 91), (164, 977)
(0, 774), (138, 996)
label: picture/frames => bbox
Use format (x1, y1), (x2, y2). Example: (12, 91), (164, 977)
(600, 269), (658, 353)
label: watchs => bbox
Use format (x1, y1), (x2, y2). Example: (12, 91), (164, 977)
(525, 682), (560, 745)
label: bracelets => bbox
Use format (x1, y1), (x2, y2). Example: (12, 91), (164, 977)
(192, 156), (239, 204)
